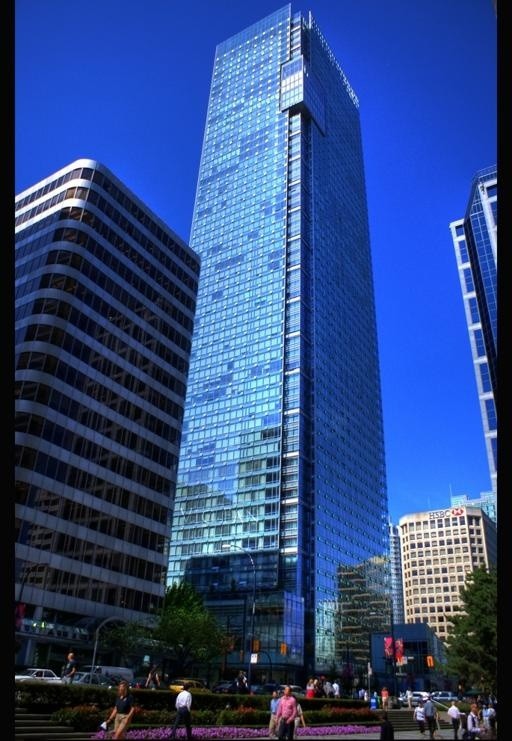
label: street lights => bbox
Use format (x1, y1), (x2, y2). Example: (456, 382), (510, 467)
(222, 544), (257, 694)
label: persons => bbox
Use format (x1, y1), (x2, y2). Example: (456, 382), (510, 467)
(62, 653), (77, 684)
(359, 687), (496, 740)
(269, 686), (305, 740)
(146, 665), (160, 690)
(306, 678), (340, 698)
(172, 683), (191, 739)
(105, 681), (135, 740)
(237, 671), (248, 688)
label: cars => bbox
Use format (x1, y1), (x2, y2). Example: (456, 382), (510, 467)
(15, 665), (307, 701)
(398, 690), (459, 706)
